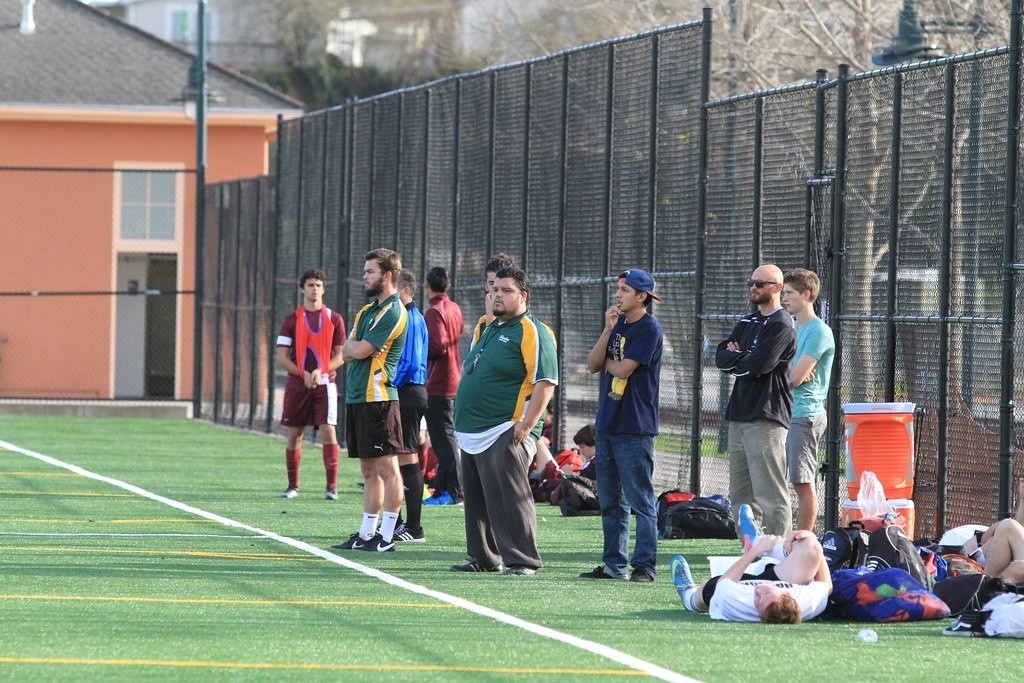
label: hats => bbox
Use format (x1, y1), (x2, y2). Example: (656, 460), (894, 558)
(618, 269), (662, 302)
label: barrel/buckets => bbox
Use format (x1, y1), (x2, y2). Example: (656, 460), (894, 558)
(840, 499), (914, 543)
(842, 404), (917, 502)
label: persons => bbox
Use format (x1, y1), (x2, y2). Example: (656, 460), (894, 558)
(356, 399), (597, 511)
(330, 247), (409, 553)
(424, 265), (463, 506)
(376, 268), (429, 543)
(961, 476), (1024, 584)
(669, 503), (834, 624)
(467, 251), (517, 355)
(715, 264), (798, 552)
(579, 269), (664, 582)
(783, 268), (835, 530)
(276, 268), (347, 500)
(449, 266), (558, 575)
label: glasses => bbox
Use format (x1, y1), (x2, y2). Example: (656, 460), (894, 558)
(746, 278), (777, 289)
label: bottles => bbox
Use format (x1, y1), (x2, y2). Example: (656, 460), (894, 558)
(857, 629), (878, 643)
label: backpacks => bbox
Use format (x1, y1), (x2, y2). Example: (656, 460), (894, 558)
(821, 521), (997, 623)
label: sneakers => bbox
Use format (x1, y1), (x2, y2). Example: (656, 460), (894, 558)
(421, 490), (456, 505)
(503, 565), (537, 576)
(942, 609), (993, 637)
(629, 567), (652, 582)
(580, 565), (628, 580)
(354, 532), (395, 552)
(393, 523), (426, 542)
(376, 519), (404, 533)
(738, 503), (767, 563)
(670, 556), (701, 612)
(331, 532), (368, 549)
(450, 559), (503, 572)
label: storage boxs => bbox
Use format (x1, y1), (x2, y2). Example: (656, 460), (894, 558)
(708, 556), (741, 578)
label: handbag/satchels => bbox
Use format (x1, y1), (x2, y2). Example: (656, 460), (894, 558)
(558, 473), (600, 516)
(657, 489), (738, 539)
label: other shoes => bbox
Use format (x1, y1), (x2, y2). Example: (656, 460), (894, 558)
(280, 487), (298, 498)
(325, 486), (338, 500)
(356, 482), (364, 489)
